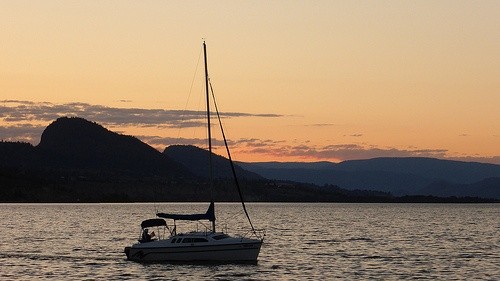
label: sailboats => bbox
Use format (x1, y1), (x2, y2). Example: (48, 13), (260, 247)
(124, 43), (265, 265)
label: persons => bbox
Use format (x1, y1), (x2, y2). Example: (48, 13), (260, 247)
(142, 229), (151, 241)
